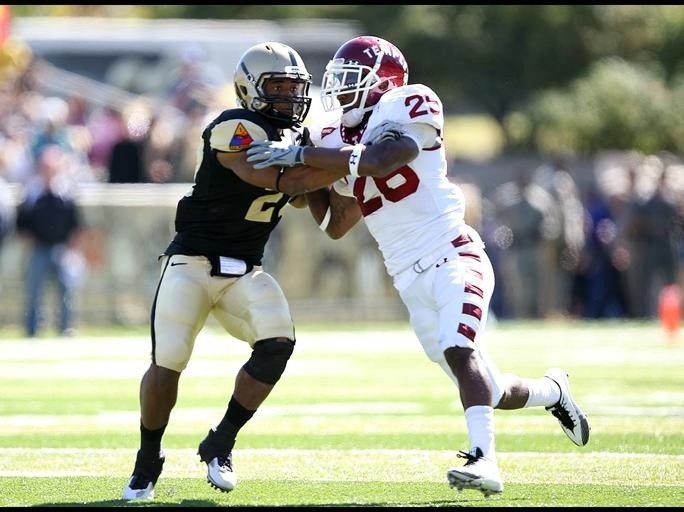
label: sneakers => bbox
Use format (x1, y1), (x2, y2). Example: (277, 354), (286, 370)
(197, 427), (236, 493)
(447, 447), (502, 497)
(544, 367), (591, 447)
(123, 449), (165, 501)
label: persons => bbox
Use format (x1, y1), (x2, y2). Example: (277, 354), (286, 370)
(120, 38), (403, 501)
(463, 152), (683, 323)
(244, 33), (592, 499)
(3, 52), (209, 342)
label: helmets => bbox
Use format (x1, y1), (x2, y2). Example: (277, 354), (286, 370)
(321, 36), (408, 129)
(234, 42), (312, 129)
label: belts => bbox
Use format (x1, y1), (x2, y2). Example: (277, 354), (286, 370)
(393, 242), (455, 292)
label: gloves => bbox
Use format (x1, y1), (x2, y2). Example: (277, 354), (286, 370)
(246, 139), (309, 170)
(362, 119), (406, 146)
